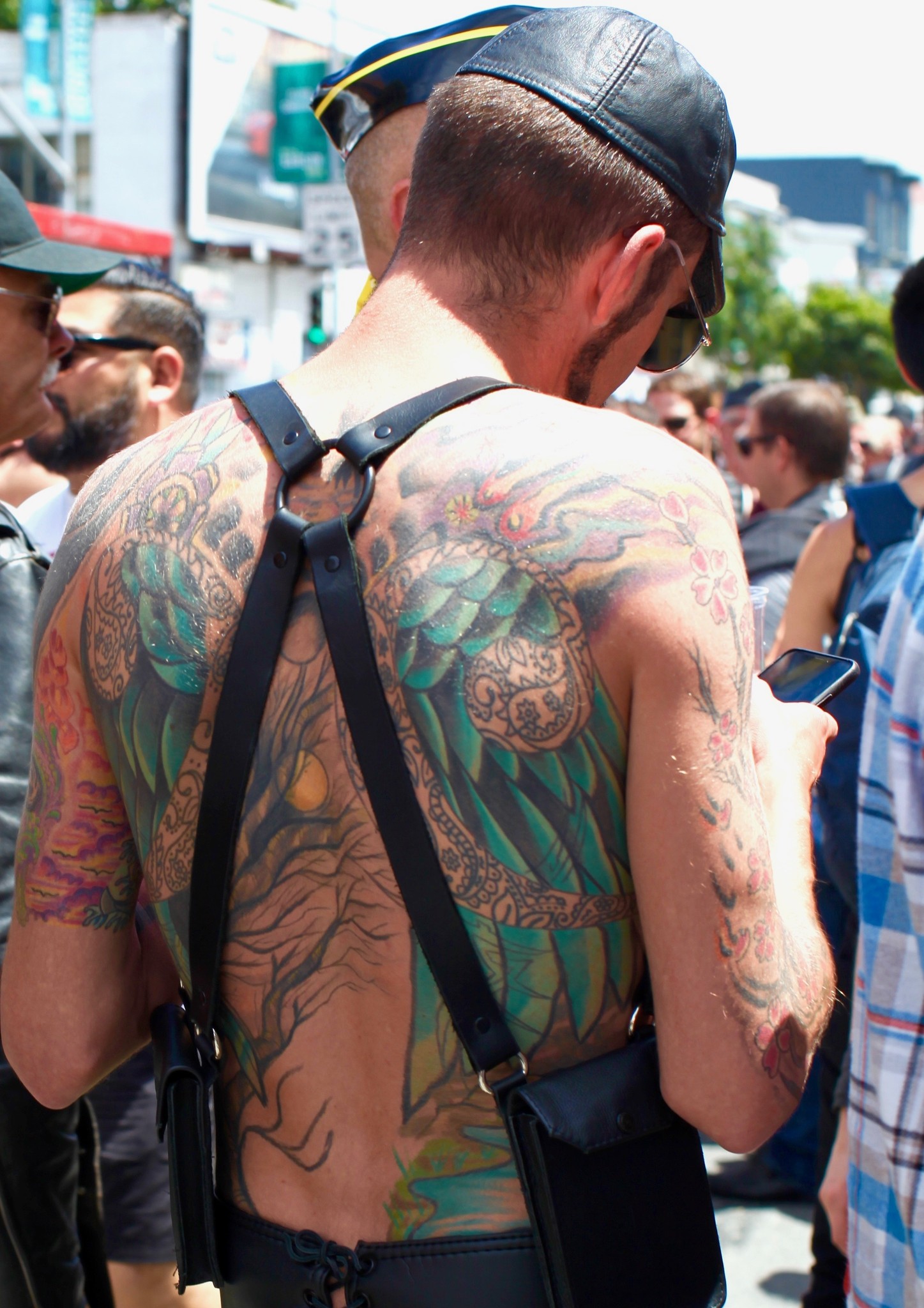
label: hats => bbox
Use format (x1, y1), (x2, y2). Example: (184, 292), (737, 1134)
(309, 5), (548, 164)
(0, 171), (123, 296)
(456, 8), (736, 318)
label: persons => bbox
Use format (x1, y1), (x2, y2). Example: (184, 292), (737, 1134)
(818, 519), (924, 1308)
(601, 376), (924, 523)
(765, 256), (924, 666)
(1, 171), (205, 1307)
(736, 379), (849, 658)
(309, 5), (547, 325)
(0, 10), (840, 1308)
(647, 373), (706, 456)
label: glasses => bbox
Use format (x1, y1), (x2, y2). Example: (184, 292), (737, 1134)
(736, 435), (795, 456)
(661, 416), (703, 431)
(623, 229), (712, 374)
(55, 335), (159, 371)
(0, 286), (63, 334)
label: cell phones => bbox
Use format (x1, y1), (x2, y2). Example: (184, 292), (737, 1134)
(756, 649), (861, 709)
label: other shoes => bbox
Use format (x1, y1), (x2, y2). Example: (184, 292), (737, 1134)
(712, 1154), (807, 1203)
